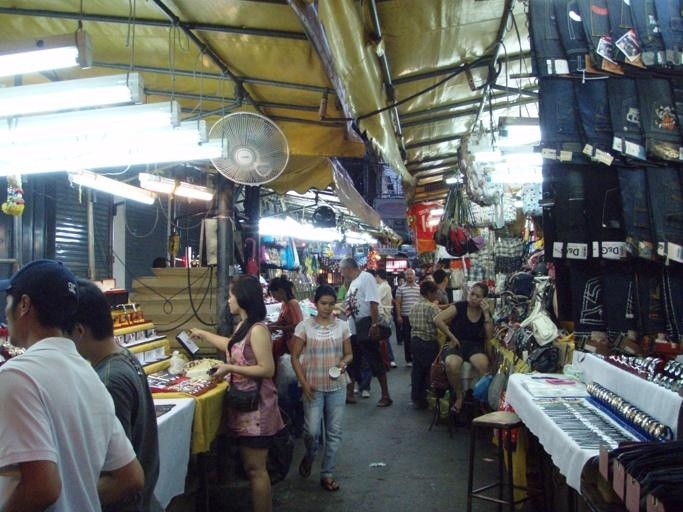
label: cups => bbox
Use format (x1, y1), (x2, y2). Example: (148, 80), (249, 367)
(328, 366), (341, 378)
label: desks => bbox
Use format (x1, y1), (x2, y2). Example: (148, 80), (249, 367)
(487, 331), (682, 512)
(149, 398), (195, 512)
(264, 298), (342, 377)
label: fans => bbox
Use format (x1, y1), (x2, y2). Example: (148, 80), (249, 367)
(206, 112), (290, 188)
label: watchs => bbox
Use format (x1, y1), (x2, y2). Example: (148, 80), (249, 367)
(531, 357), (683, 450)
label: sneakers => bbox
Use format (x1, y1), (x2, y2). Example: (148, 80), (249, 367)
(345, 362), (424, 409)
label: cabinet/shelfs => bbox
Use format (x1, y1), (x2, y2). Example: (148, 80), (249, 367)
(100, 295), (229, 512)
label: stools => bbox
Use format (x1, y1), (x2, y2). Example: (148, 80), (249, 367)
(464, 410), (548, 512)
(427, 374), (464, 437)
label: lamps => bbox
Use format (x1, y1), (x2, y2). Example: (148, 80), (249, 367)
(0, 2), (229, 204)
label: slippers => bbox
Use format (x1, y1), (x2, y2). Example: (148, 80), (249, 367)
(320, 474), (338, 490)
(299, 456), (313, 478)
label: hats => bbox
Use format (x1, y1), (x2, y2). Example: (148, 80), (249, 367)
(0, 258), (79, 308)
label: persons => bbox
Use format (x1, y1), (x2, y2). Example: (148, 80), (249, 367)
(187, 273), (285, 512)
(291, 283), (354, 492)
(0, 258), (145, 512)
(265, 274), (304, 354)
(336, 257), (450, 411)
(433, 282), (494, 416)
(71, 279), (164, 512)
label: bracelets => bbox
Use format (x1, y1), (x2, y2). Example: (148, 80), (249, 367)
(483, 320), (491, 323)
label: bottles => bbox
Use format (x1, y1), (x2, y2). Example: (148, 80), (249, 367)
(189, 255), (199, 266)
(184, 244), (193, 267)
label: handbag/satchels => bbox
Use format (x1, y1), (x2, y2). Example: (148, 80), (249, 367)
(355, 316), (374, 346)
(223, 370), (263, 412)
(495, 312), (560, 373)
(430, 345), (449, 391)
(433, 218), (485, 257)
